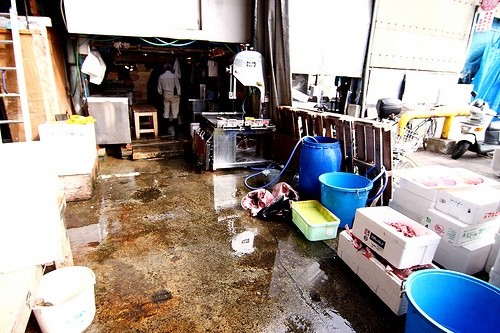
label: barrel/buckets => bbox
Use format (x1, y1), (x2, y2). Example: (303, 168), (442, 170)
(261, 162), (281, 189)
(299, 135), (342, 200)
(400, 269), (500, 333)
(318, 172), (374, 228)
(29, 266), (97, 333)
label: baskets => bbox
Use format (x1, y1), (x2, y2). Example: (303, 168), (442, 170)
(392, 127), (422, 152)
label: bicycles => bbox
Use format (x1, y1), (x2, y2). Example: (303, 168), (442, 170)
(390, 102), (446, 160)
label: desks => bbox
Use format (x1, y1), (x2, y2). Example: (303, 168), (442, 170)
(188, 98), (208, 121)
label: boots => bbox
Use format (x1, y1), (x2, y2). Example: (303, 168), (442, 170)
(172, 117), (178, 132)
(163, 118), (169, 131)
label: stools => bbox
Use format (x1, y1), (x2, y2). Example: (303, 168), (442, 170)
(131, 103), (159, 140)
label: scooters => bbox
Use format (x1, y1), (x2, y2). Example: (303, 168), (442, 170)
(300, 96), (402, 153)
(452, 91), (500, 159)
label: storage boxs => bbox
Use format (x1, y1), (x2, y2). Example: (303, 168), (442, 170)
(391, 183), (436, 219)
(484, 231), (500, 273)
(350, 205), (443, 270)
(424, 208), (498, 248)
(493, 250), (500, 276)
(38, 118), (97, 177)
(433, 227), (500, 275)
(486, 267), (500, 289)
(397, 165), (493, 202)
(387, 199), (424, 225)
(437, 182), (500, 225)
(290, 200), (341, 241)
(337, 228), (440, 316)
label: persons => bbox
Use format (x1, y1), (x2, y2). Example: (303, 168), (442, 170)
(459, 36), (500, 118)
(157, 65), (181, 125)
